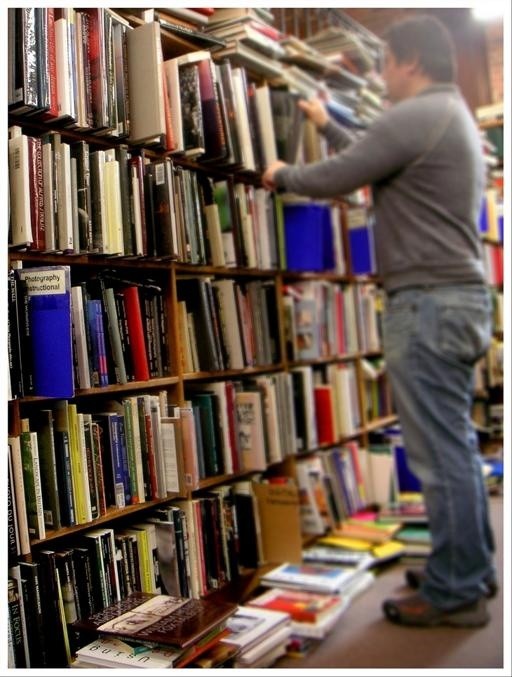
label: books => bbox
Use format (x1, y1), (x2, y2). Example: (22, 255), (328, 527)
(9, 8), (502, 667)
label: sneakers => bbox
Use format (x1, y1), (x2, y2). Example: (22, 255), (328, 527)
(379, 590), (491, 628)
(404, 562), (499, 599)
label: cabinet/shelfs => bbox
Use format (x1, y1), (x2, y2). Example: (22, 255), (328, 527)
(469, 113), (502, 494)
(8, 10), (398, 668)
